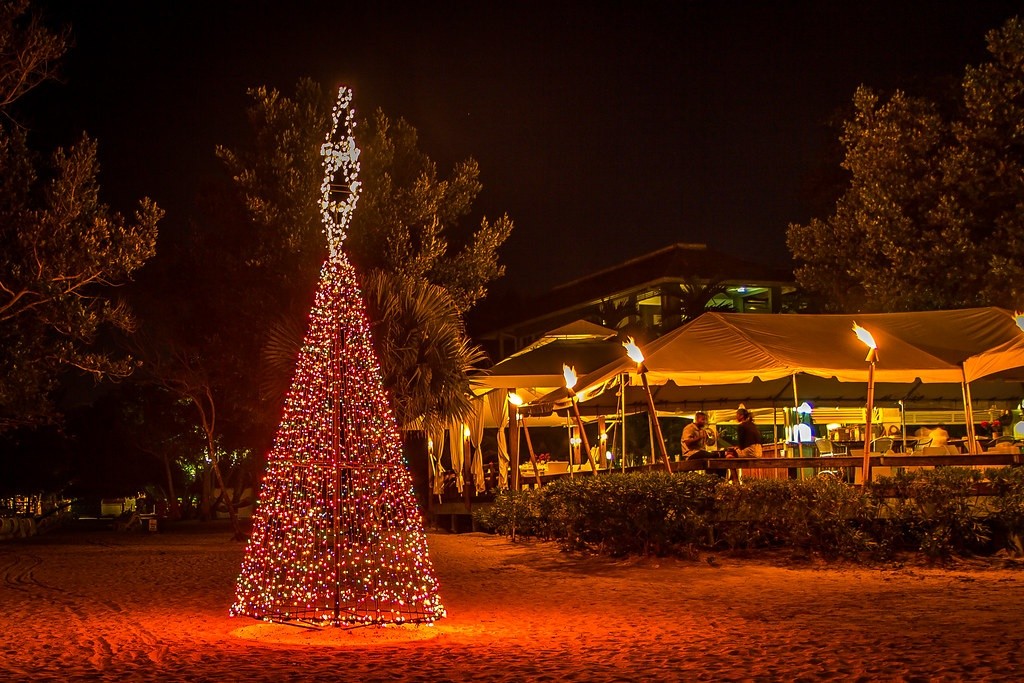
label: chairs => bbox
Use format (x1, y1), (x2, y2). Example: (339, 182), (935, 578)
(814, 438), (848, 479)
(869, 436), (894, 452)
(547, 461), (569, 474)
(985, 435), (1015, 448)
(913, 437), (933, 452)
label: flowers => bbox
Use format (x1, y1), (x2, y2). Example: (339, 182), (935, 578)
(981, 420), (1001, 436)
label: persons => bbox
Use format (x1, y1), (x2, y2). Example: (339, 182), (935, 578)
(680, 411), (727, 477)
(723, 408), (763, 485)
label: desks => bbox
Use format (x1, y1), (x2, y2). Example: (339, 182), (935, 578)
(762, 438), (1024, 458)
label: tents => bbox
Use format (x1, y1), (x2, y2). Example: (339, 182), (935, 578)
(398, 306), (1024, 541)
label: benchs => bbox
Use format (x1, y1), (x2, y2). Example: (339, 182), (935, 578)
(524, 455), (1024, 496)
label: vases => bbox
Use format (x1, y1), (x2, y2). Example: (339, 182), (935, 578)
(992, 432), (1000, 440)
